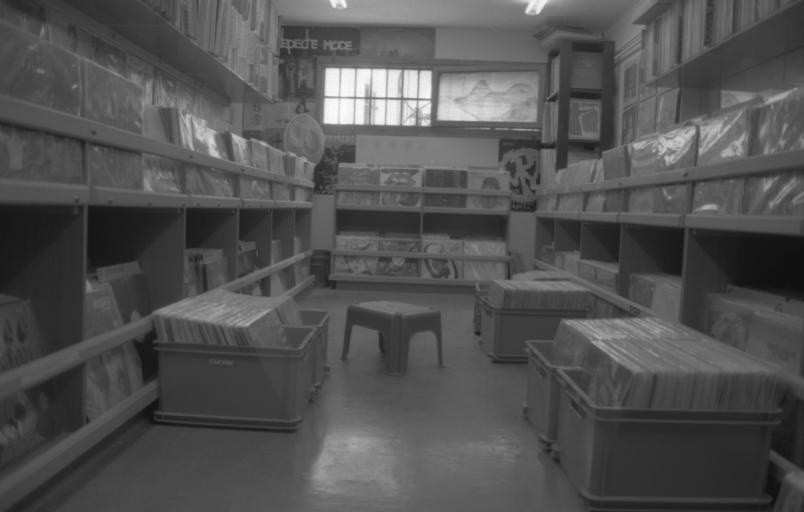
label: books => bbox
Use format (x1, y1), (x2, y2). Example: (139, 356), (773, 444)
(486, 279), (787, 411)
(333, 163), (509, 281)
(0, 2), (312, 453)
(537, 2), (803, 385)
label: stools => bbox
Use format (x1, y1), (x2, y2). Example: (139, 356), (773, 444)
(340, 299), (447, 377)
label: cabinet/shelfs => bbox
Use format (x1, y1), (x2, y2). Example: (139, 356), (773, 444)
(328, 158), (515, 294)
(0, 0), (324, 511)
(534, 0), (804, 512)
